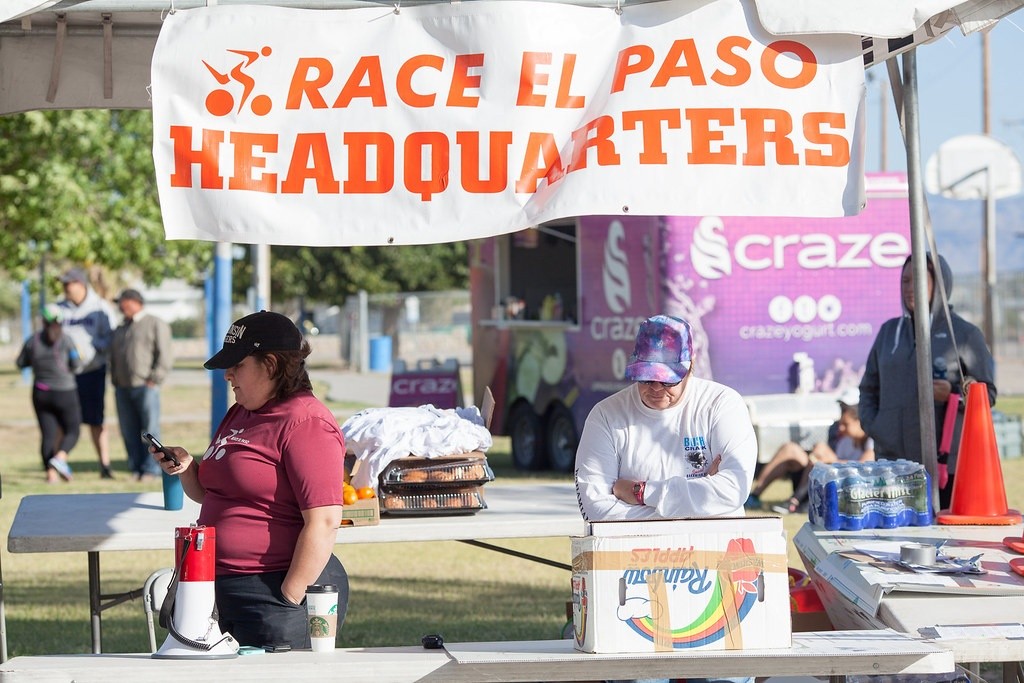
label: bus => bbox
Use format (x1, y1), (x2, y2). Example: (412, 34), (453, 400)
(342, 285), (478, 368)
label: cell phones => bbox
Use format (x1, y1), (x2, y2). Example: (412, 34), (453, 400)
(422, 635), (443, 648)
(141, 433), (180, 466)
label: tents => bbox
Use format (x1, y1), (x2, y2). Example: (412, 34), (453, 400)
(1, 0), (1020, 508)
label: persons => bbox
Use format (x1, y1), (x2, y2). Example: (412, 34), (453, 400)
(858, 251), (996, 507)
(744, 402), (875, 515)
(47, 268), (123, 483)
(16, 302), (83, 485)
(110, 289), (173, 488)
(574, 316), (759, 521)
(148, 310), (349, 652)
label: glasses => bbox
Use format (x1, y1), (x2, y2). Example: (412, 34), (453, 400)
(638, 380), (682, 387)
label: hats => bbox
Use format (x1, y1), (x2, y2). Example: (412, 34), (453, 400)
(204, 310), (302, 370)
(834, 387), (860, 406)
(625, 314), (694, 383)
(113, 290), (144, 303)
(55, 269), (88, 290)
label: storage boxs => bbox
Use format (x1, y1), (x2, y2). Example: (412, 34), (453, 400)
(572, 515), (792, 654)
(340, 492), (379, 528)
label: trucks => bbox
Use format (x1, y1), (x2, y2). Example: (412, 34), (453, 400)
(468, 167), (913, 476)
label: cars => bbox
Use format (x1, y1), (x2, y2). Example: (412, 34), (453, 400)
(117, 279), (204, 338)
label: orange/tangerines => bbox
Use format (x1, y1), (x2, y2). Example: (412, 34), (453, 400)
(341, 469), (375, 524)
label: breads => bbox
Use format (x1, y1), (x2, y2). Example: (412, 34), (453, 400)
(385, 465), (484, 509)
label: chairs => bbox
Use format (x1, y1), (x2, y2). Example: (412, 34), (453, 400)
(142, 567), (173, 655)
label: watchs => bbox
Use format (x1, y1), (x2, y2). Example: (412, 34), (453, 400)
(632, 479), (646, 505)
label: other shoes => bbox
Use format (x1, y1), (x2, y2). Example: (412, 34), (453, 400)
(743, 495), (762, 509)
(771, 495), (802, 514)
(101, 464), (113, 478)
(129, 472), (157, 482)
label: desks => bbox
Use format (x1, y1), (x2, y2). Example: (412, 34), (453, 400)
(6, 473), (589, 656)
(0, 629), (955, 683)
(794, 514), (1024, 683)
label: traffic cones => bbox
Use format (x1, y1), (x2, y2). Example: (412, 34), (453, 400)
(936, 381), (1024, 526)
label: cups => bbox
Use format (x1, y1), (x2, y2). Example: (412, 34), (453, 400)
(162, 469), (183, 510)
(305, 584), (338, 652)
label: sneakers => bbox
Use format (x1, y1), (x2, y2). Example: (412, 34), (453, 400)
(48, 457), (74, 481)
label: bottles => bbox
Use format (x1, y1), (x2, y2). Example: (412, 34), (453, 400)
(808, 458), (933, 531)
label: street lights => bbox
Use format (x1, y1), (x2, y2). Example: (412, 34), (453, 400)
(34, 240), (50, 317)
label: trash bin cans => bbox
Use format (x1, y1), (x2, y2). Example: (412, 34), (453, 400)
(368, 334), (393, 371)
